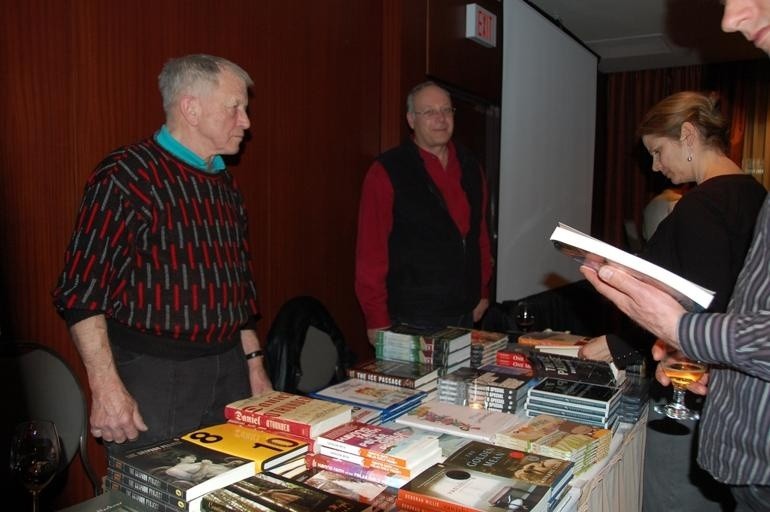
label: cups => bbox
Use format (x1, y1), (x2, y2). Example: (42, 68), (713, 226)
(515, 301), (537, 331)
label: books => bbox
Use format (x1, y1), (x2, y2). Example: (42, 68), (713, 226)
(547, 219), (719, 314)
(59, 318), (660, 512)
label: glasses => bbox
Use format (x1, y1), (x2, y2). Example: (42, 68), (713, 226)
(414, 107), (456, 120)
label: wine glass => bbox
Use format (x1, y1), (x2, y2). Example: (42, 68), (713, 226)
(8, 419), (62, 512)
(653, 342), (709, 422)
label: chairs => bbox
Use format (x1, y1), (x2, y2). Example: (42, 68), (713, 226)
(252, 292), (346, 396)
(0, 343), (85, 512)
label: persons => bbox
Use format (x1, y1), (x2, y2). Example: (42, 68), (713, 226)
(352, 81), (498, 350)
(641, 187), (689, 245)
(577, 2), (769, 511)
(47, 54), (280, 463)
(573, 87), (768, 372)
